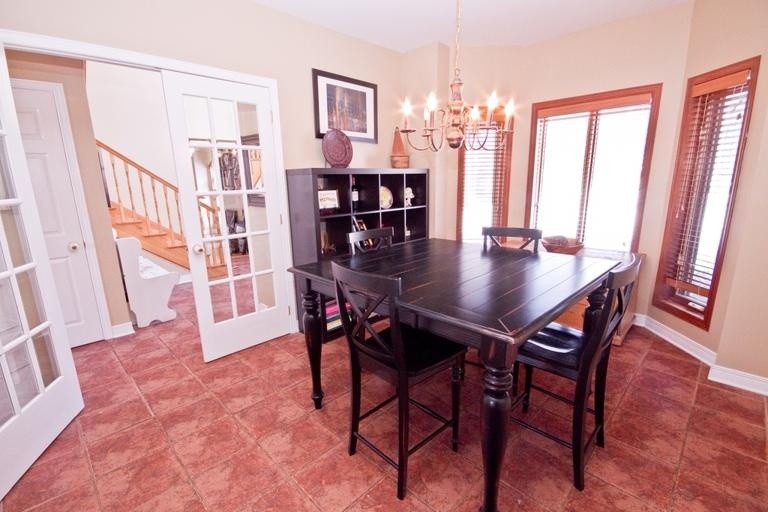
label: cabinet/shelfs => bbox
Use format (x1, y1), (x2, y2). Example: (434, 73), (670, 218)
(285, 167), (431, 345)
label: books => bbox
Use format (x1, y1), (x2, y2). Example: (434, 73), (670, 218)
(326, 298), (379, 331)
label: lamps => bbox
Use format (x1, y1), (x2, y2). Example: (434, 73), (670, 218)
(397, 1), (516, 154)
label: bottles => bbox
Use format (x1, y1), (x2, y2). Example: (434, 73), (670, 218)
(351, 176), (360, 211)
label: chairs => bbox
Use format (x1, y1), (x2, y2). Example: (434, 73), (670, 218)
(330, 226), (648, 491)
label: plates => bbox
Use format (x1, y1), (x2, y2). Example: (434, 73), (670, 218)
(321, 128), (353, 169)
(379, 186), (394, 209)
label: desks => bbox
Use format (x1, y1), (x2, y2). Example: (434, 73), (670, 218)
(286, 237), (626, 511)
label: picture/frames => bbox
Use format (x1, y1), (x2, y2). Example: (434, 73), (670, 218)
(240, 133), (266, 209)
(311, 68), (378, 145)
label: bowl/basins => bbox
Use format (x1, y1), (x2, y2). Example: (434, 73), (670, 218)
(540, 236), (585, 255)
